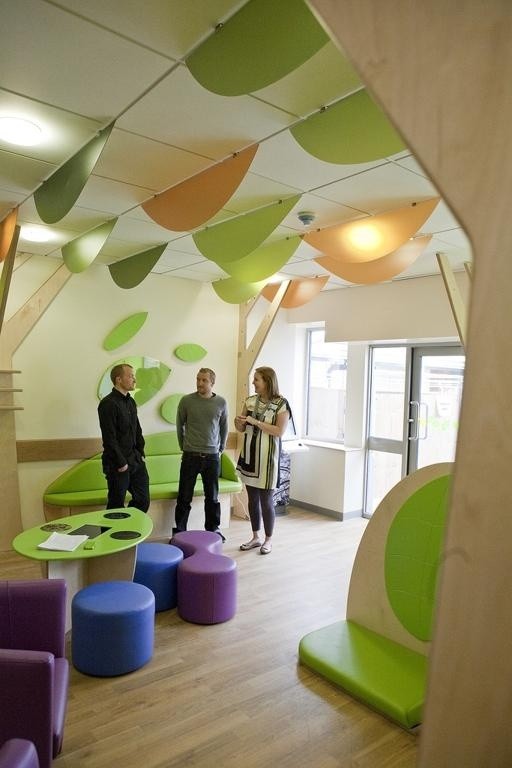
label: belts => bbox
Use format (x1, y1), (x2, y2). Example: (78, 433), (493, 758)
(183, 451), (221, 458)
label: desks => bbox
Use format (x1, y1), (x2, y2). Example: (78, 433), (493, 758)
(12, 507), (153, 635)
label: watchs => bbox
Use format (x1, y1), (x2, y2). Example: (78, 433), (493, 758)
(256, 421), (261, 426)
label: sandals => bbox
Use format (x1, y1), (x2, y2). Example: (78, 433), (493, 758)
(260, 543), (273, 553)
(240, 541), (264, 550)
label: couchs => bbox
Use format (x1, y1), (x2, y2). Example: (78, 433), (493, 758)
(0, 579), (70, 768)
(0, 738), (40, 768)
(43, 431), (243, 542)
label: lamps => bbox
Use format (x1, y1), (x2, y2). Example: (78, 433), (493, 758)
(21, 224), (53, 243)
(0, 116), (43, 146)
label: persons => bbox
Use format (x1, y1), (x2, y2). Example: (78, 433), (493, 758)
(234, 367), (291, 555)
(170, 368), (226, 543)
(98, 363), (150, 534)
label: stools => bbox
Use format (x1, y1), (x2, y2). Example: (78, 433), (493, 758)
(170, 530), (237, 625)
(133, 542), (184, 613)
(71, 581), (155, 678)
